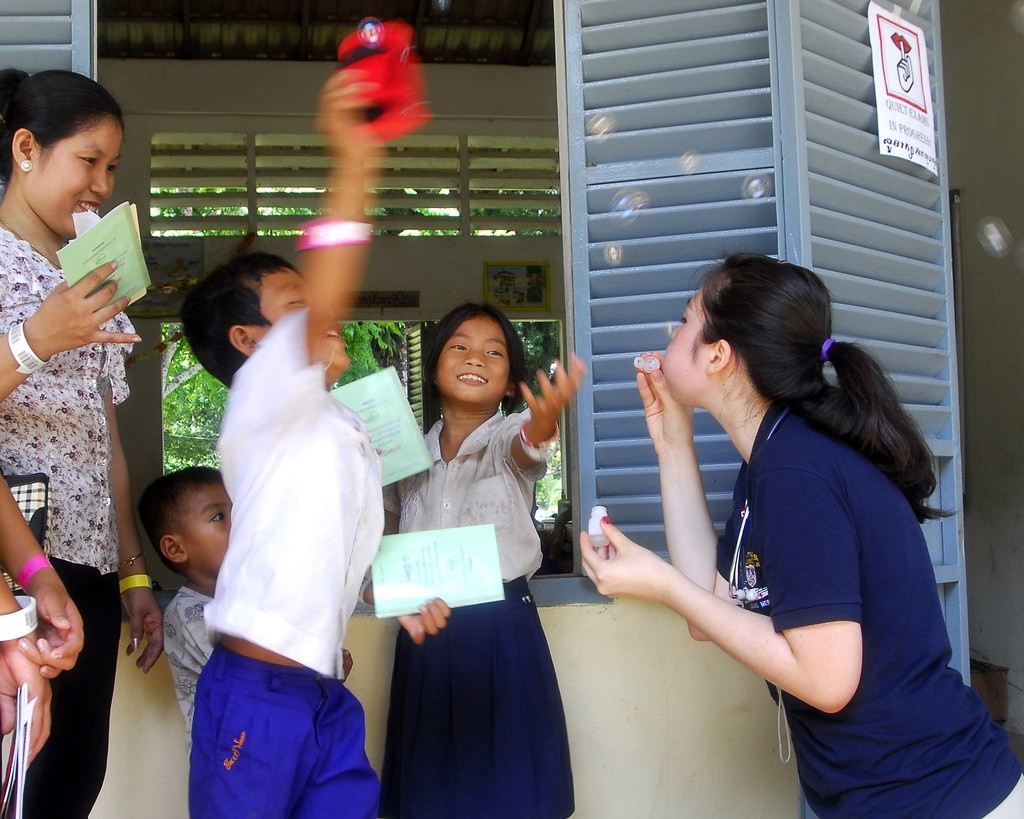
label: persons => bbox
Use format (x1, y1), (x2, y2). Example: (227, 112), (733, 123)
(139, 64), (588, 819)
(0, 65), (165, 819)
(575, 251), (1024, 819)
(0, 473), (85, 773)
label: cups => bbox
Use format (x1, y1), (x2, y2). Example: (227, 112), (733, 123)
(334, 22), (431, 143)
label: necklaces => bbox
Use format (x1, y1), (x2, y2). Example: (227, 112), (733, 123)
(722, 404), (792, 611)
(0, 218), (62, 270)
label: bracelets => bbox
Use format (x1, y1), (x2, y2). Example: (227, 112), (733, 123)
(295, 218), (372, 247)
(0, 595), (38, 642)
(119, 573), (153, 593)
(117, 552), (145, 569)
(514, 419), (561, 464)
(15, 554), (54, 590)
(9, 322), (51, 377)
